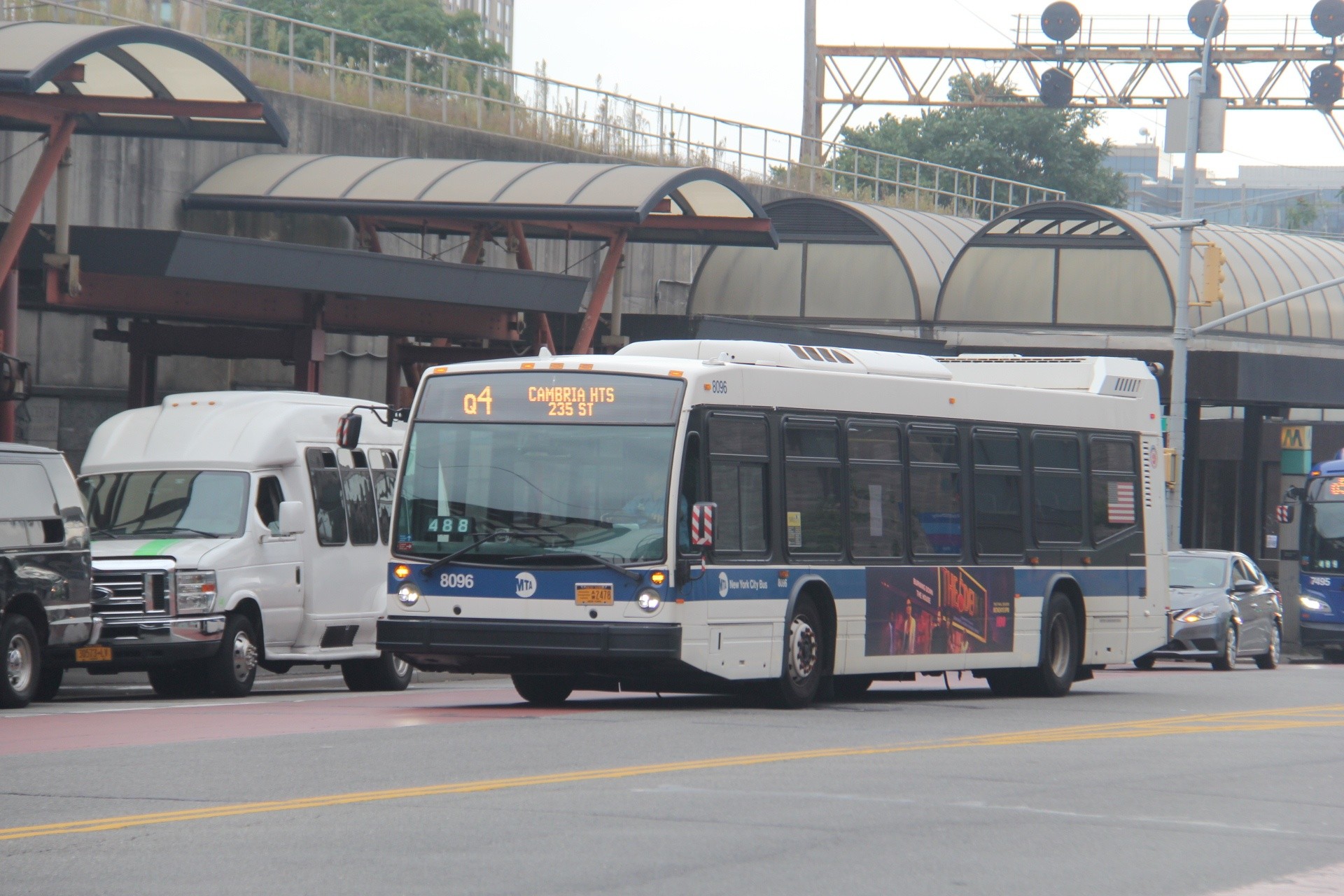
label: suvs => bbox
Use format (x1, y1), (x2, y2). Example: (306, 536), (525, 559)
(0, 442), (93, 710)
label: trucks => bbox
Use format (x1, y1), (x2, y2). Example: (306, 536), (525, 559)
(76, 389), (418, 695)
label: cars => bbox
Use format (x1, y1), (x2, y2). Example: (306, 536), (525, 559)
(1131, 549), (1283, 671)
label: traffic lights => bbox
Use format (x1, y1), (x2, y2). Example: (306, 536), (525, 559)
(1203, 246), (1227, 302)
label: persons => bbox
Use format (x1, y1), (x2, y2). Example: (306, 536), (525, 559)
(604, 467), (691, 554)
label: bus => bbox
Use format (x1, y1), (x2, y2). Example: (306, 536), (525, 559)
(1274, 448), (1343, 664)
(337, 337), (1167, 709)
